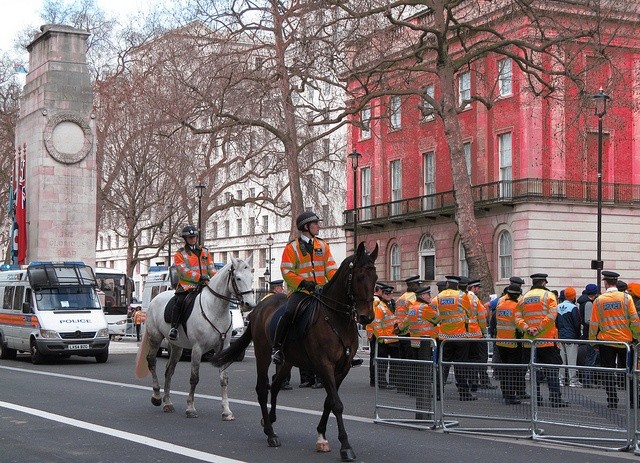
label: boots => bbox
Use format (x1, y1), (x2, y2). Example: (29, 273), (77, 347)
(232, 330), (237, 336)
(272, 316), (292, 364)
(169, 306), (182, 341)
(351, 359), (363, 366)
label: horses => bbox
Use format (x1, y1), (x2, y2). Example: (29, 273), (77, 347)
(134, 252), (257, 421)
(209, 239), (378, 462)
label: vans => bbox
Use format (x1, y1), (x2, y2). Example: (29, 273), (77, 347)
(0, 261), (110, 364)
(139, 262), (246, 360)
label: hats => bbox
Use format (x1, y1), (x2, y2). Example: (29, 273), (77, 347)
(436, 281), (446, 286)
(375, 282), (387, 288)
(445, 276), (461, 283)
(631, 284), (640, 297)
(467, 280), (483, 287)
(560, 290), (564, 298)
(602, 271), (620, 280)
(509, 277), (525, 286)
(530, 274), (548, 283)
(458, 276), (469, 286)
(380, 286), (394, 294)
(507, 285), (522, 293)
(616, 281), (627, 291)
(586, 284), (597, 295)
(564, 288), (576, 301)
(415, 286), (431, 295)
(403, 275), (423, 284)
(268, 280), (284, 286)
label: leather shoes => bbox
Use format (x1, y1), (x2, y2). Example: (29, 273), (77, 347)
(284, 385), (292, 390)
(471, 381), (478, 392)
(505, 397), (521, 404)
(300, 381), (312, 388)
(537, 401), (544, 406)
(480, 382), (498, 389)
(460, 394), (477, 401)
(516, 393), (530, 399)
(370, 383), (375, 386)
(312, 383), (323, 389)
(552, 402), (569, 408)
(379, 382), (395, 390)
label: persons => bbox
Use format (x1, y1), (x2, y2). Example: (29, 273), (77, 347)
(509, 276), (525, 302)
(134, 307), (141, 341)
(560, 290), (566, 302)
(365, 283), (387, 389)
(392, 275), (422, 396)
(273, 213), (363, 366)
(496, 285), (530, 405)
(466, 279), (498, 391)
(616, 280), (628, 292)
(269, 280), (293, 390)
(429, 280), (447, 385)
(577, 284), (602, 387)
(459, 276), (469, 294)
(168, 226), (238, 339)
(482, 287), (509, 380)
(589, 272), (640, 408)
(370, 286), (401, 389)
(513, 274), (568, 407)
(627, 282), (640, 369)
(408, 286), (441, 397)
(554, 288), (583, 387)
(551, 290), (558, 304)
(429, 276), (477, 402)
(299, 369), (321, 389)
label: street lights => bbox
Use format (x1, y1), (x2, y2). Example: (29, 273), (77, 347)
(267, 235), (274, 281)
(348, 148), (362, 251)
(264, 267), (270, 294)
(591, 89), (612, 293)
(195, 181), (205, 244)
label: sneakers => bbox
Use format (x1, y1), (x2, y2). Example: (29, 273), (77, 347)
(584, 383), (600, 388)
(560, 380), (565, 386)
(569, 381), (582, 388)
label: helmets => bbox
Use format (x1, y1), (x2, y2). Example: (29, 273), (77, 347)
(296, 212), (323, 231)
(180, 226), (199, 237)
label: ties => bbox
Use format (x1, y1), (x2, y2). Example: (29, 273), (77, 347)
(306, 238), (313, 252)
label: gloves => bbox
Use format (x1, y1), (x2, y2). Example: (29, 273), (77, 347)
(299, 280), (315, 292)
(200, 274), (210, 281)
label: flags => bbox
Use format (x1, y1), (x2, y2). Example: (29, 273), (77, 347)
(8, 174), (18, 266)
(15, 161), (26, 265)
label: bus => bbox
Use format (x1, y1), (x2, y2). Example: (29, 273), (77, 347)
(96, 267), (134, 341)
